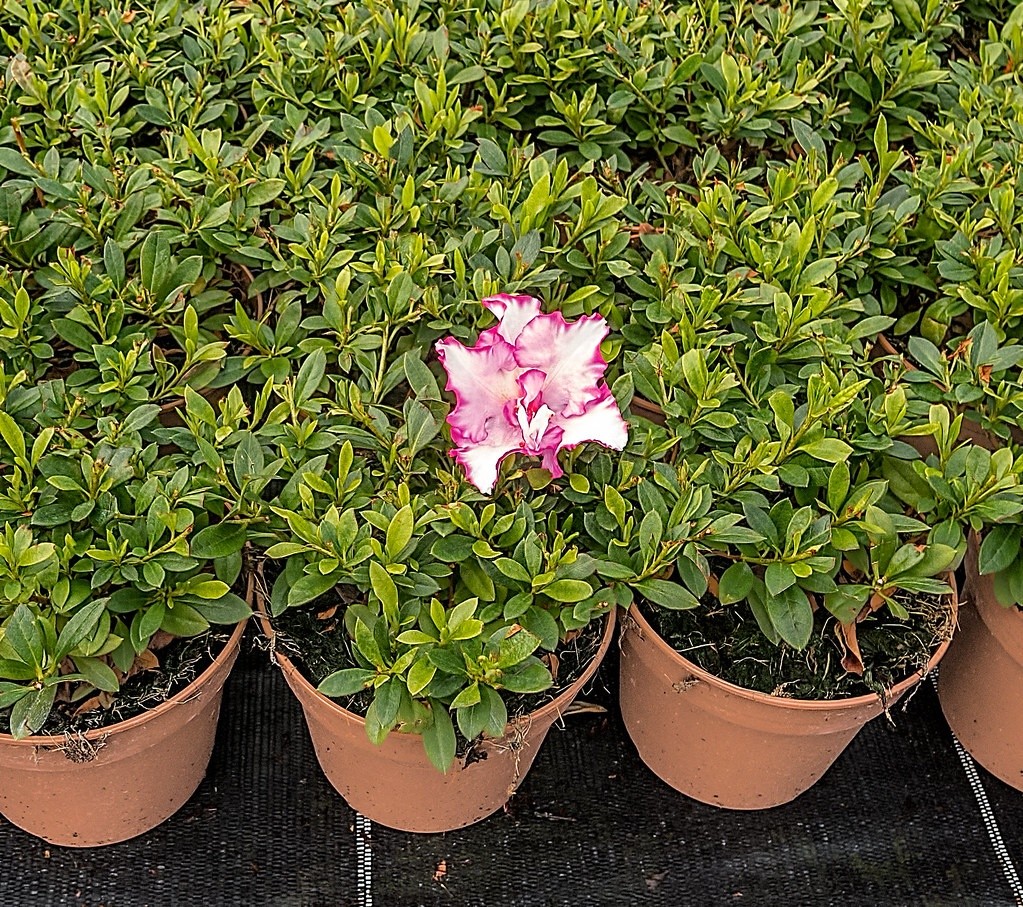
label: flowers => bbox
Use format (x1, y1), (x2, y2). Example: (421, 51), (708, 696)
(433, 291), (632, 495)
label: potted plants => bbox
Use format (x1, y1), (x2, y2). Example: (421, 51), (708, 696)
(0, 0), (1023, 849)
